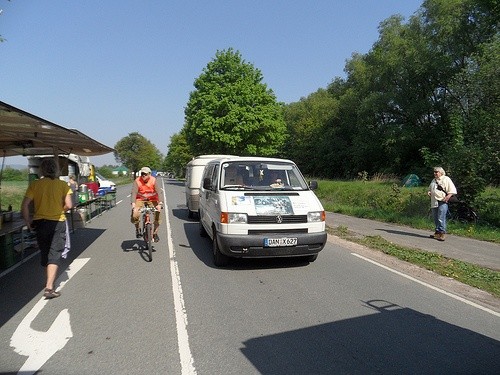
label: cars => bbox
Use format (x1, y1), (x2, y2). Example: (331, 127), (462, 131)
(94, 172), (117, 196)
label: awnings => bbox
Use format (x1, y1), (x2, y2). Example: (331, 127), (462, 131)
(0, 101), (117, 157)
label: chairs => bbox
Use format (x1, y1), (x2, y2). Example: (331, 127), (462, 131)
(223, 167), (244, 186)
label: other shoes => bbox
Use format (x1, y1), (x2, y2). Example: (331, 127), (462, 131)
(153, 233), (159, 243)
(136, 228), (141, 238)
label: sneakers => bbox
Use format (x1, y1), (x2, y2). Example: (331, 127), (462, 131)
(439, 234), (445, 241)
(43, 289), (60, 298)
(430, 232), (439, 238)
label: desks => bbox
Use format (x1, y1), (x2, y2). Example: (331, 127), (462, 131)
(0, 189), (116, 278)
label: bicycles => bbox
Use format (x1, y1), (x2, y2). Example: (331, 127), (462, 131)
(131, 205), (164, 262)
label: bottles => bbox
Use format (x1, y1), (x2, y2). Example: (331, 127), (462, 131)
(8, 204), (12, 212)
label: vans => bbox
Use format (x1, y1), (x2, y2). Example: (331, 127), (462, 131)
(198, 158), (327, 267)
(185, 155), (239, 218)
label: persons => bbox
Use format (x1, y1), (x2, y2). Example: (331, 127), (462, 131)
(21, 159), (74, 299)
(131, 166), (164, 243)
(427, 167), (458, 242)
(270, 174), (290, 188)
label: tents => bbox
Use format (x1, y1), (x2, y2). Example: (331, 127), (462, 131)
(402, 174), (423, 188)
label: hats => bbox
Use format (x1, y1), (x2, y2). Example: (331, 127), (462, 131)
(141, 167), (151, 174)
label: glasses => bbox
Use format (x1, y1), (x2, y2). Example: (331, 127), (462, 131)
(434, 171), (438, 173)
